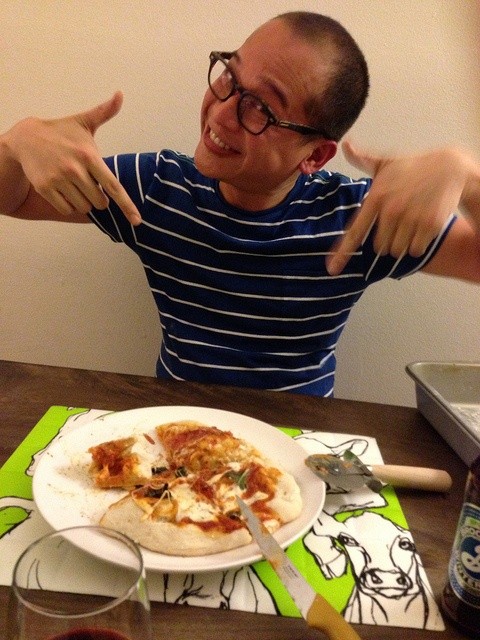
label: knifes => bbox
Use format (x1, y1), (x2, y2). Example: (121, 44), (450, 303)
(234, 494), (365, 639)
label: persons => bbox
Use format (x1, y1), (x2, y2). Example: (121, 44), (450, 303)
(0, 12), (480, 397)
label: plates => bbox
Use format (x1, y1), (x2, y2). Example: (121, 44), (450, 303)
(31, 405), (329, 575)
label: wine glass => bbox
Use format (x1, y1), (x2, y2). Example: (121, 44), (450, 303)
(6, 524), (153, 640)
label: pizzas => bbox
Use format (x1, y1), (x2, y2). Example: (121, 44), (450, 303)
(64, 421), (302, 558)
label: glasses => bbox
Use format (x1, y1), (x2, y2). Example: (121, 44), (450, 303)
(207, 52), (340, 142)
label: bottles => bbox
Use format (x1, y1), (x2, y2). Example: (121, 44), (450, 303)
(437, 455), (480, 635)
